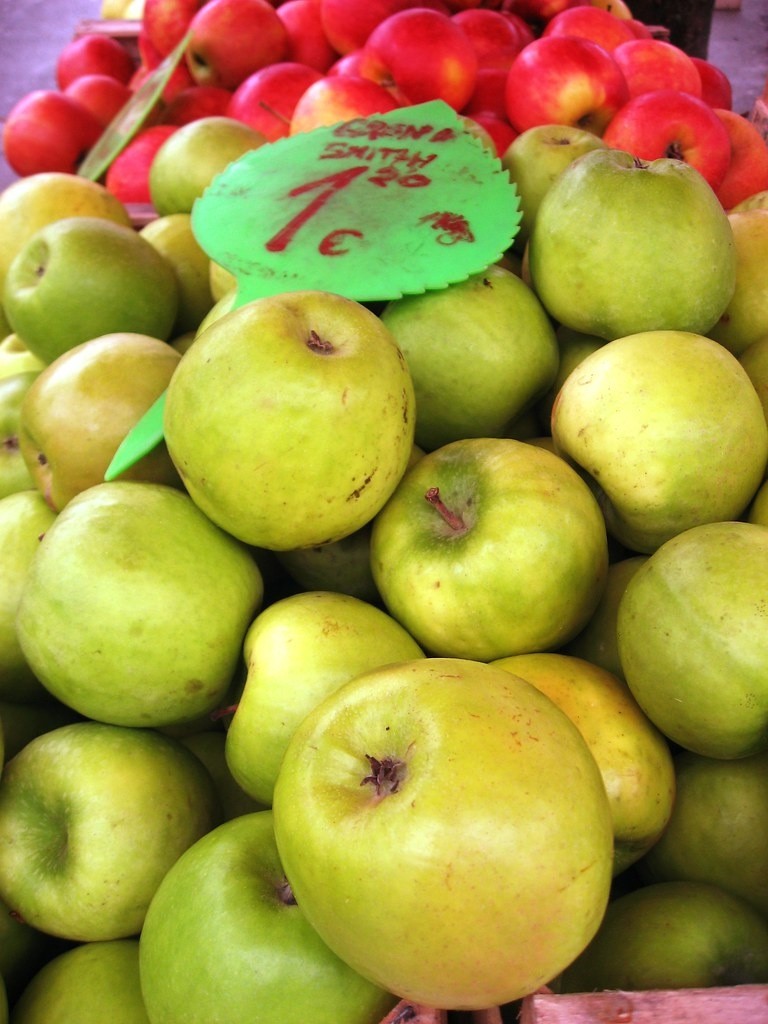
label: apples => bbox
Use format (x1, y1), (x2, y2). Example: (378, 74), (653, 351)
(0, 0), (768, 1024)
